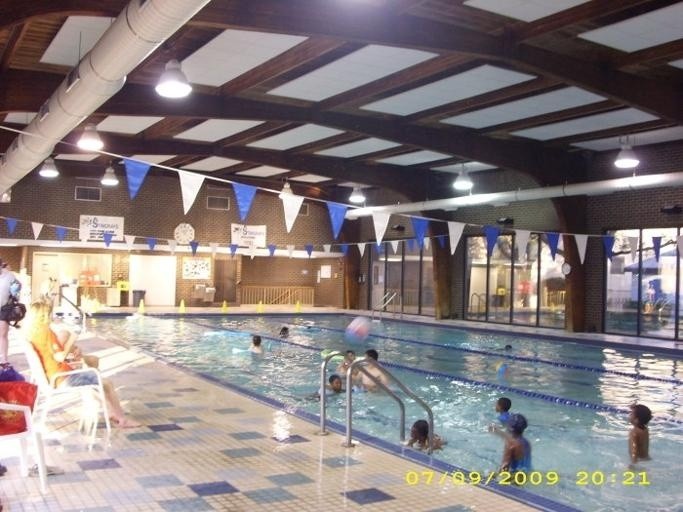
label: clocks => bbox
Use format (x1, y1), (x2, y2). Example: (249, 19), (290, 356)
(174, 223), (195, 245)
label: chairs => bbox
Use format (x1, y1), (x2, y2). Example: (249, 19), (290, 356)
(20, 338), (112, 433)
(1, 367), (51, 494)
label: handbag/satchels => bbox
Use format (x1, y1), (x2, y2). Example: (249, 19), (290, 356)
(0, 295), (25, 326)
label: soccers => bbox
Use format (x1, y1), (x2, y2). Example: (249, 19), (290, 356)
(496, 362), (510, 378)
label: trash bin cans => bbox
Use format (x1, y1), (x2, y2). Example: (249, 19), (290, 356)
(133, 290), (146, 307)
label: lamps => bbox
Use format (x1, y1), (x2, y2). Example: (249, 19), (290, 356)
(349, 183), (366, 203)
(77, 117), (104, 151)
(452, 162), (473, 190)
(154, 59), (192, 98)
(279, 177), (295, 200)
(38, 156), (59, 178)
(101, 159), (119, 186)
(613, 134), (640, 169)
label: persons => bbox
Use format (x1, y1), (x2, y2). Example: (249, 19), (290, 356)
(488, 398), (531, 481)
(683, 317), (683, 320)
(247, 335), (263, 354)
(0, 261), (142, 431)
(279, 326), (290, 338)
(411, 420), (441, 452)
(318, 374), (347, 396)
(356, 349), (380, 393)
(335, 351), (355, 393)
(491, 397), (511, 422)
(629, 404), (653, 465)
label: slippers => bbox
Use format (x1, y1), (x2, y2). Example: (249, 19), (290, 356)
(112, 419), (140, 427)
